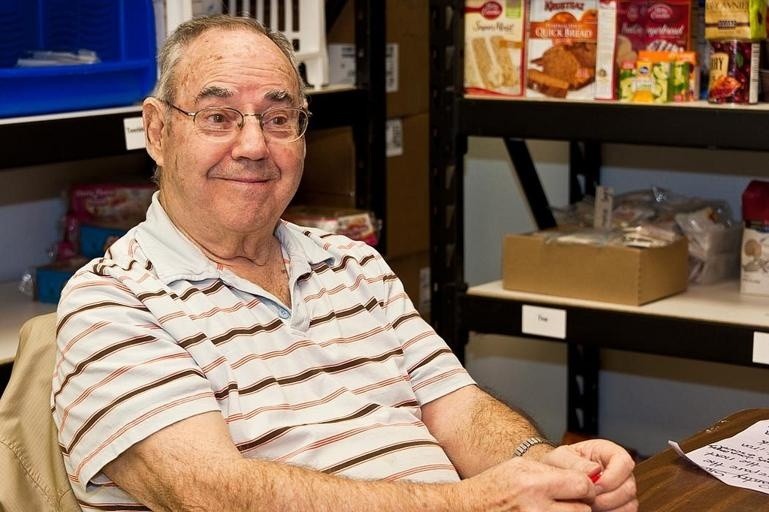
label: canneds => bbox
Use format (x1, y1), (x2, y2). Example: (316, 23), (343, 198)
(708, 38), (761, 105)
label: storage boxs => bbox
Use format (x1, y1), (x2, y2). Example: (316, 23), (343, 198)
(502, 231), (689, 307)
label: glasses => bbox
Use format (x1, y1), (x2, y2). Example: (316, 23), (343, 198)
(160, 100), (312, 145)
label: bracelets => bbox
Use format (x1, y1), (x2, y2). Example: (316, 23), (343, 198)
(512, 436), (559, 459)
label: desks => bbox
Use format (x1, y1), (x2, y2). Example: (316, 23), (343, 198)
(635, 407), (769, 511)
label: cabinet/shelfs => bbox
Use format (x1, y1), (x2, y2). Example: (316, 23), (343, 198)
(1, 0), (386, 370)
(425, 1), (768, 439)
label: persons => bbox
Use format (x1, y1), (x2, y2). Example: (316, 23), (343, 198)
(44, 14), (643, 511)
